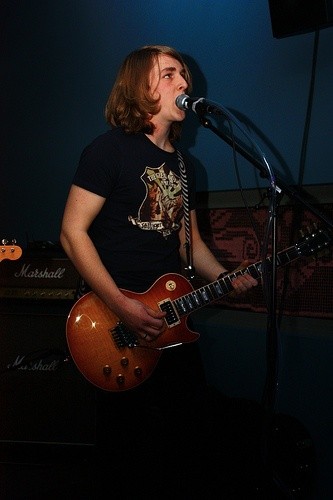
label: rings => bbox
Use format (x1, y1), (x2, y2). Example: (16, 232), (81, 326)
(142, 333), (147, 340)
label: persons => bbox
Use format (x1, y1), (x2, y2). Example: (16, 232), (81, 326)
(56, 44), (259, 500)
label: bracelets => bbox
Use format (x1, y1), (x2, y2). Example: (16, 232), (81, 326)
(216, 271), (229, 280)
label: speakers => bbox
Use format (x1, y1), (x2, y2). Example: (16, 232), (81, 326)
(1, 303), (106, 454)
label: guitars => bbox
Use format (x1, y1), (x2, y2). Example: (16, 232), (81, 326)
(66, 222), (332, 395)
(0, 239), (22, 263)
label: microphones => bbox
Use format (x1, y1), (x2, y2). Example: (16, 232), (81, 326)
(175, 94), (227, 118)
